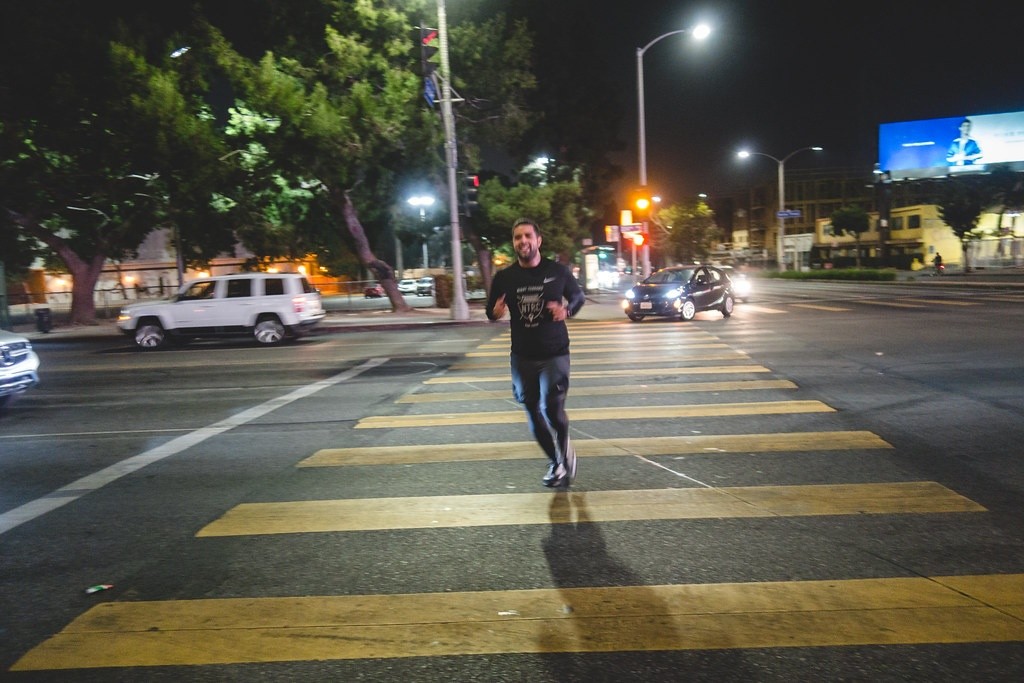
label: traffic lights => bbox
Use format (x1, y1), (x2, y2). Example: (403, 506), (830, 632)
(408, 25), (440, 76)
(458, 170), (480, 219)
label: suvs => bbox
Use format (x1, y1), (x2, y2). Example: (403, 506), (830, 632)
(115, 272), (326, 353)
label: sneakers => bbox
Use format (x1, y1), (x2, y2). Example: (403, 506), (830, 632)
(541, 451), (578, 486)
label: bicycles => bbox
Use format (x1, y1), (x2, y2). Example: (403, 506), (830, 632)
(930, 260), (946, 277)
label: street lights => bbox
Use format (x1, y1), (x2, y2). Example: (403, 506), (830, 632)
(637, 24), (710, 282)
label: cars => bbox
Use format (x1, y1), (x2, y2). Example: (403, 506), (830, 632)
(417, 277), (433, 296)
(363, 283), (387, 298)
(708, 247), (810, 273)
(716, 265), (750, 304)
(0, 328), (41, 406)
(397, 280), (417, 295)
(736, 146), (823, 265)
(622, 264), (735, 321)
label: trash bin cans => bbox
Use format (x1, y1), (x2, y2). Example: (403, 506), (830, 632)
(434, 274), (452, 309)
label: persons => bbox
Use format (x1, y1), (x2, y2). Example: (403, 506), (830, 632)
(485, 217), (587, 488)
